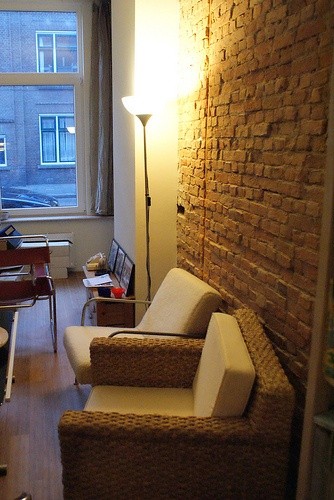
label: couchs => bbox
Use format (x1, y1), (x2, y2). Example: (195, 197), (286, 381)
(59, 309), (294, 500)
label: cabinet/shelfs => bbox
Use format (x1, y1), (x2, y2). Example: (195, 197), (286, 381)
(83, 266), (132, 327)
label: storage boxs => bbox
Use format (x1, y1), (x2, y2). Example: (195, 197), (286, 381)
(47, 240), (74, 280)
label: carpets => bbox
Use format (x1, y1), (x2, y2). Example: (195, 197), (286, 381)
(0, 308), (17, 406)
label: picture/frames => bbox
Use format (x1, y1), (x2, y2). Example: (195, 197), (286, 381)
(107, 238), (135, 297)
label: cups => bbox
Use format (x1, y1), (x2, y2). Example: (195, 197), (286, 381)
(112, 287), (125, 299)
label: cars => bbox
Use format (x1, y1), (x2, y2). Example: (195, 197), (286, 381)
(0, 187), (61, 210)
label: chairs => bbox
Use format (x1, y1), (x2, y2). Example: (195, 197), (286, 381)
(63, 268), (224, 385)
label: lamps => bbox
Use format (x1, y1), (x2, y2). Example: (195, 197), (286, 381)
(122, 95), (167, 309)
(66, 127), (76, 134)
